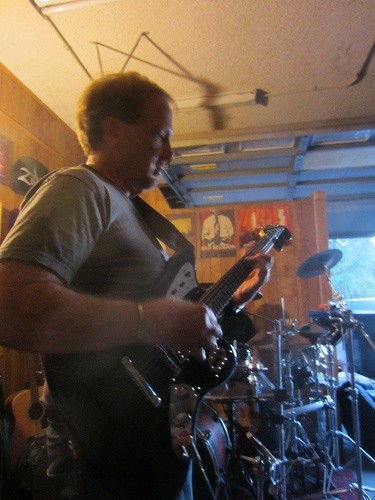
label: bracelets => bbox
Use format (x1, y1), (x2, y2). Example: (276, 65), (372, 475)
(135, 300), (146, 343)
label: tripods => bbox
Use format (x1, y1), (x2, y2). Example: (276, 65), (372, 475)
(265, 270), (375, 500)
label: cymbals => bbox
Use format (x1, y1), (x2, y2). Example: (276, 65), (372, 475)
(297, 249), (343, 278)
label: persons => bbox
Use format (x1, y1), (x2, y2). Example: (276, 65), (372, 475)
(0, 70), (272, 500)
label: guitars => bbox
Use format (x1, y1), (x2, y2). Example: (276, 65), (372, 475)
(6, 350), (43, 470)
(42, 225), (293, 465)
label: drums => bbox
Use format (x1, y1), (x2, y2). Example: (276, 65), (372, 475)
(194, 403), (231, 500)
(14, 435), (44, 500)
(259, 344), (331, 391)
